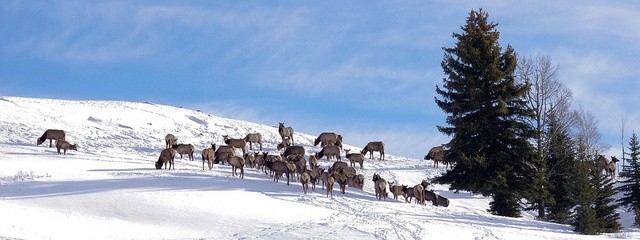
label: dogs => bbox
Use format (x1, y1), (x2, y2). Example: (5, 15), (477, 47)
(301, 170), (311, 193)
(326, 173), (334, 198)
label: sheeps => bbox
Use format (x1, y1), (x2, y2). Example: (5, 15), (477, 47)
(388, 181), (406, 199)
(609, 156), (620, 177)
(267, 160), (290, 185)
(215, 146), (235, 158)
(309, 155), (317, 170)
(155, 148), (177, 170)
(37, 130), (65, 146)
(263, 152), (280, 175)
(401, 184), (413, 202)
(431, 151), (455, 169)
(427, 189), (449, 207)
(296, 154), (306, 180)
(281, 146), (304, 160)
(361, 141), (385, 160)
(372, 173), (387, 200)
(278, 122), (294, 146)
(314, 132), (337, 146)
(254, 151), (263, 170)
(56, 140), (77, 155)
(353, 174), (364, 191)
(172, 144), (194, 161)
(316, 147), (340, 162)
(165, 134), (178, 148)
(240, 133), (262, 151)
(243, 153), (254, 168)
(333, 173), (347, 194)
(202, 143), (216, 170)
(339, 167), (355, 178)
(344, 149), (364, 168)
(315, 164), (325, 184)
(227, 152), (245, 179)
(413, 180), (430, 204)
(214, 151), (233, 164)
(222, 135), (245, 157)
(327, 162), (346, 173)
(597, 154), (608, 176)
